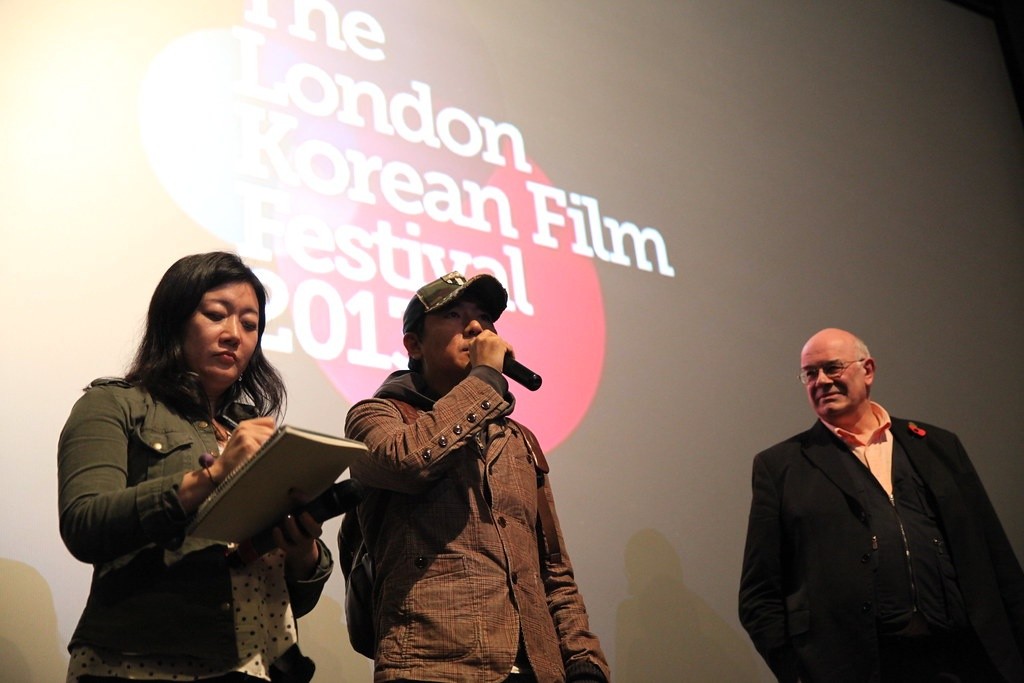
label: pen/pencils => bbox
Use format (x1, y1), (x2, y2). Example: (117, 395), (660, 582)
(221, 414), (240, 429)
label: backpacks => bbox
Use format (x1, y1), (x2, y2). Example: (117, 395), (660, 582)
(337, 396), (562, 660)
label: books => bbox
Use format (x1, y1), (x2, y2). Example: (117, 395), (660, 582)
(187, 426), (367, 544)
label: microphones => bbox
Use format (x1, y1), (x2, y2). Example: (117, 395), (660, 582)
(227, 478), (364, 571)
(503, 355), (543, 391)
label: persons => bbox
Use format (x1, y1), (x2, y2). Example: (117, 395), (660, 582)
(739, 327), (1024, 683)
(56, 253), (334, 682)
(344, 271), (611, 683)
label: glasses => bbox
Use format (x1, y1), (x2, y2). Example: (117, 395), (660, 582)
(797, 358), (863, 385)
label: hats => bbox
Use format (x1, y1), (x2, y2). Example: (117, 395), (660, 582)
(402, 271), (509, 335)
